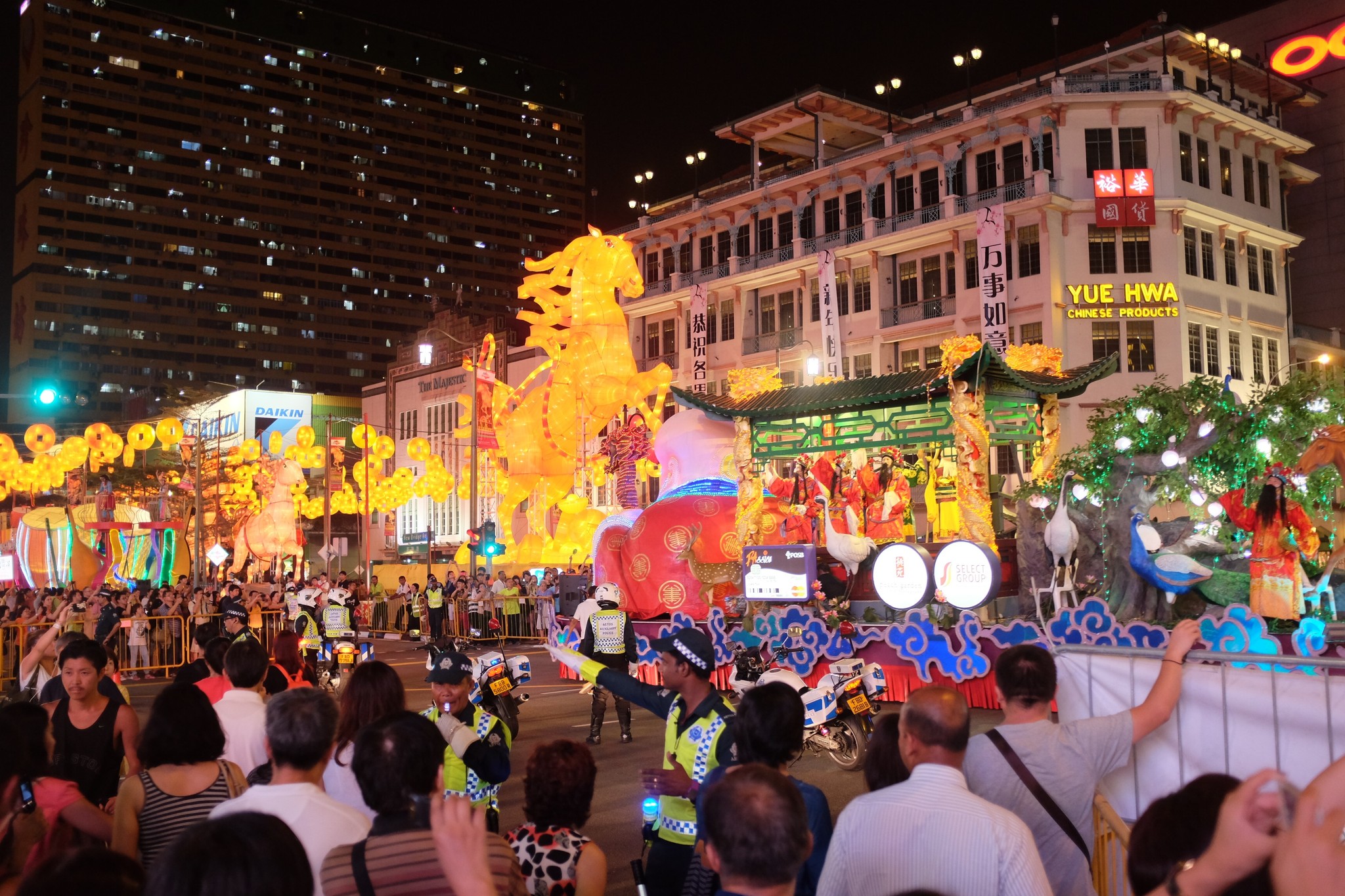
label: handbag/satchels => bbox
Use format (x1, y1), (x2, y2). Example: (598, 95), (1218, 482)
(0, 663), (40, 707)
(471, 585), (478, 599)
(477, 606), (484, 614)
(152, 624), (169, 642)
(542, 600), (549, 617)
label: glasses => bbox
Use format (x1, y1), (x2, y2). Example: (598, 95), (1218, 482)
(23, 610), (31, 613)
(120, 596), (128, 600)
(164, 594), (175, 597)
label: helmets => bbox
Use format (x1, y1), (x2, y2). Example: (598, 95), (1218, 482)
(297, 587), (323, 610)
(327, 587), (352, 607)
(595, 581), (620, 607)
(285, 581), (295, 592)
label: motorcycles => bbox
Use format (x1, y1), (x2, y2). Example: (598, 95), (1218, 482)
(725, 625), (888, 771)
(318, 610), (376, 701)
(412, 617), (531, 741)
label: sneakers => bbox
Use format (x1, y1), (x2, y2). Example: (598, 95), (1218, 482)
(585, 733), (601, 744)
(620, 731), (633, 743)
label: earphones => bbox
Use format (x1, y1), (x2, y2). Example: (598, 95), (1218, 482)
(233, 620), (237, 623)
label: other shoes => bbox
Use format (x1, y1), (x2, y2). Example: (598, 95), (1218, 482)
(444, 634), (542, 647)
(120, 670), (178, 682)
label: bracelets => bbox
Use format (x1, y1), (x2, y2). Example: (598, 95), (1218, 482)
(682, 780), (696, 799)
(1162, 857), (1196, 896)
(1162, 659), (1186, 665)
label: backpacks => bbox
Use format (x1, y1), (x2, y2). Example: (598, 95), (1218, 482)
(269, 661), (313, 690)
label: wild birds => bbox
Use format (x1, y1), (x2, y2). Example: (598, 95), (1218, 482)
(1128, 504), (1251, 628)
(1044, 469), (1086, 624)
(806, 494), (880, 601)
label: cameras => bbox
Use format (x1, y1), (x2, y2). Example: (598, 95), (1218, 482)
(201, 593), (211, 599)
(18, 778), (36, 815)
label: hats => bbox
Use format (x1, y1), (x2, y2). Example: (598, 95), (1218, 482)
(578, 564), (589, 571)
(430, 577), (437, 582)
(793, 453), (815, 471)
(217, 602), (249, 620)
(868, 445), (906, 466)
(569, 569), (576, 574)
(832, 453), (853, 471)
(93, 589), (112, 598)
(649, 626), (716, 676)
(424, 651), (472, 684)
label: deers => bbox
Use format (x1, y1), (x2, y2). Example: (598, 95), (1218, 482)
(675, 522), (754, 618)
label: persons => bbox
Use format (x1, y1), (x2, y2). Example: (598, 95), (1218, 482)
(1123, 755), (1345, 896)
(543, 627), (743, 896)
(960, 618), (1205, 896)
(694, 681), (835, 895)
(146, 808), (318, 896)
(1133, 784), (1345, 896)
(702, 762), (815, 895)
(811, 683), (1056, 896)
(1217, 461), (1322, 637)
(14, 843), (149, 896)
(105, 681), (251, 896)
(319, 706), (532, 896)
(204, 686), (377, 896)
(863, 712), (915, 792)
(1, 562), (597, 810)
(765, 443), (961, 544)
(501, 737), (611, 896)
(575, 581), (641, 747)
(0, 697), (124, 896)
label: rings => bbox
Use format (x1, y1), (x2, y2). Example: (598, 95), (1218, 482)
(654, 777), (657, 782)
(654, 784), (657, 789)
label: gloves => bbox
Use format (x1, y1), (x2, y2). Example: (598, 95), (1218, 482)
(542, 642), (610, 689)
(435, 712), (482, 760)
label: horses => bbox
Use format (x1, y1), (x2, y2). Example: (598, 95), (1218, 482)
(466, 222), (673, 564)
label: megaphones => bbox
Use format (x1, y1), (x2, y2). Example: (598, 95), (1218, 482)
(420, 615), (427, 622)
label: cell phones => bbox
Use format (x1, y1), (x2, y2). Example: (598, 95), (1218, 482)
(63, 598), (66, 601)
(450, 560), (456, 564)
(101, 586), (103, 589)
(234, 581), (240, 585)
(556, 583), (559, 587)
(470, 582), (475, 588)
(15, 585), (20, 587)
(186, 582), (190, 585)
(550, 569), (555, 571)
(485, 574), (490, 577)
(42, 607), (47, 610)
(588, 555), (592, 557)
(1277, 781), (1300, 836)
(177, 594), (180, 599)
(483, 586), (485, 589)
(459, 572), (466, 576)
(72, 603), (86, 613)
(207, 586), (213, 590)
(37, 588), (40, 590)
(261, 594), (266, 597)
